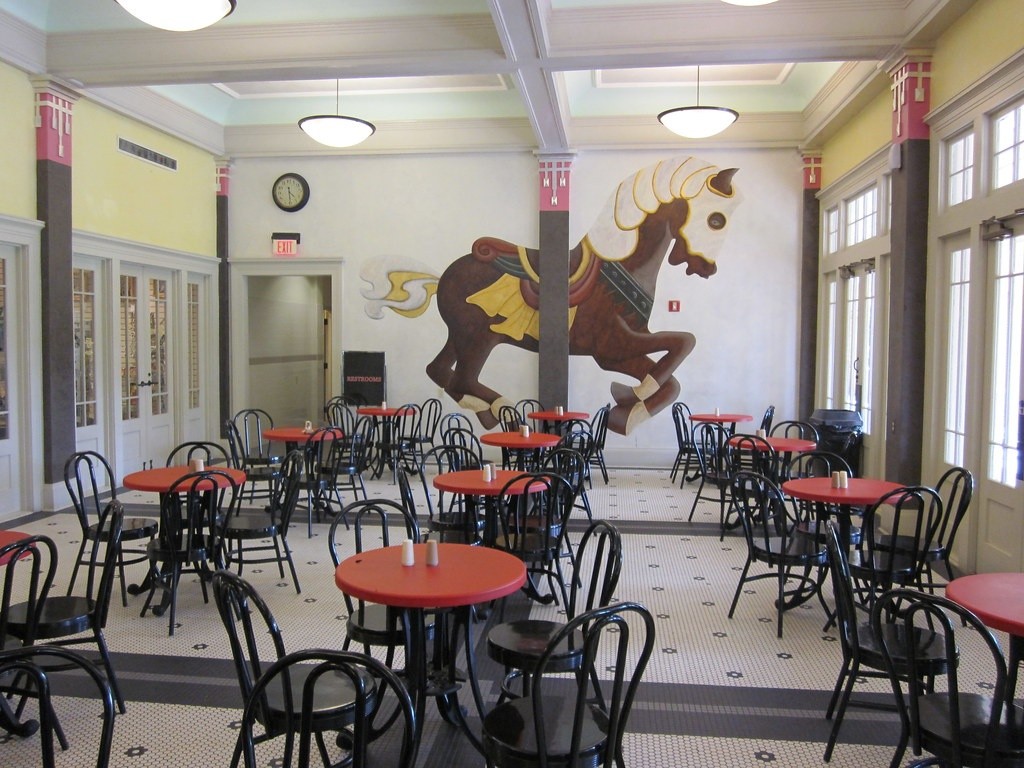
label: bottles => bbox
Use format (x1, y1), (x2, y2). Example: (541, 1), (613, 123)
(520, 425), (529, 437)
(715, 407), (719, 416)
(555, 406), (563, 415)
(305, 421), (311, 432)
(425, 540), (438, 567)
(189, 459), (204, 475)
(483, 463), (496, 482)
(756, 429), (766, 441)
(381, 401), (387, 410)
(402, 538), (414, 566)
(833, 471), (848, 489)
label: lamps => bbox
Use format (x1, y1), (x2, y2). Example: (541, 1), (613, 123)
(296, 78), (376, 148)
(657, 66), (741, 140)
(113, 0), (237, 33)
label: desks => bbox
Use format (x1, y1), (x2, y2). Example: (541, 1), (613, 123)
(332, 539), (530, 753)
(528, 408), (589, 438)
(946, 571), (1024, 706)
(0, 530), (40, 738)
(429, 466), (553, 607)
(355, 405), (418, 481)
(776, 473), (920, 613)
(121, 464), (246, 617)
(722, 435), (817, 531)
(686, 412), (753, 483)
(478, 432), (564, 472)
(262, 424), (344, 520)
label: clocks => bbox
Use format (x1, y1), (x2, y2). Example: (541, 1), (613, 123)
(271, 173), (310, 212)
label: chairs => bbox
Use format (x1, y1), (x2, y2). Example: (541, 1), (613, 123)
(0, 393), (1024, 768)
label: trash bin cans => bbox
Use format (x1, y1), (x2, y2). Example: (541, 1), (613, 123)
(808, 408), (864, 478)
